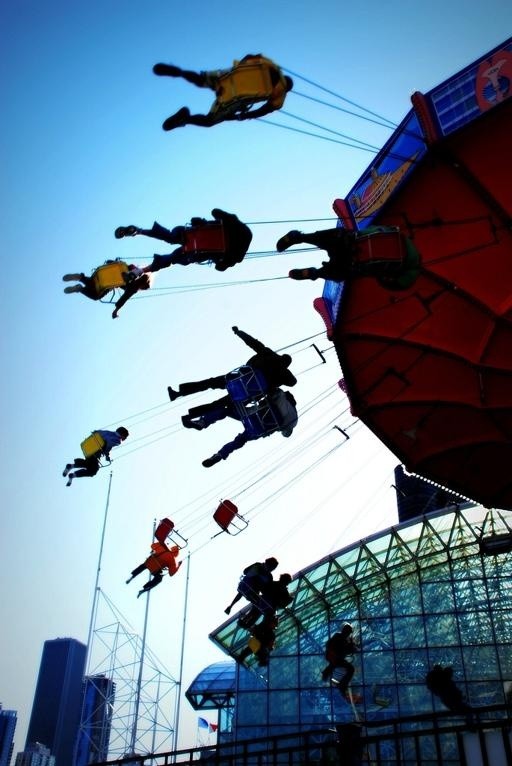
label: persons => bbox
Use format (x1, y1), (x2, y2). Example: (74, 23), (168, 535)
(152, 53), (294, 131)
(113, 207), (253, 282)
(424, 665), (482, 734)
(224, 557), (279, 615)
(238, 574), (296, 628)
(61, 427), (128, 487)
(182, 388), (299, 467)
(236, 616), (280, 666)
(125, 540), (182, 599)
(275, 225), (423, 290)
(61, 259), (152, 319)
(321, 623), (357, 698)
(167, 326), (297, 423)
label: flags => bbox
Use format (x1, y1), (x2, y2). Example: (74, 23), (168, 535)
(205, 722), (217, 733)
(197, 716), (208, 728)
